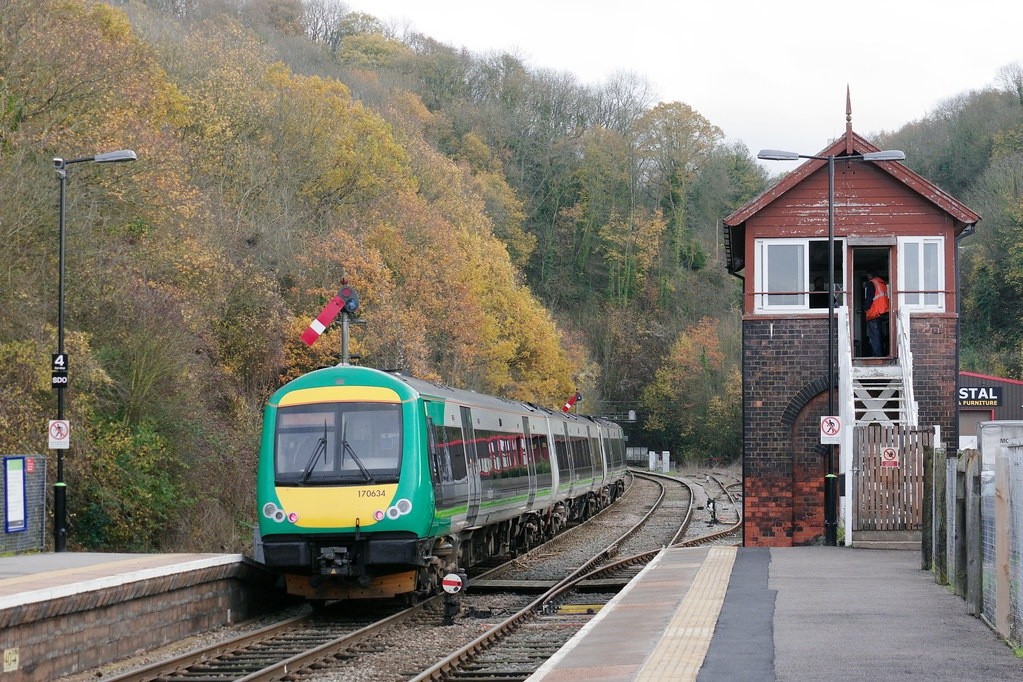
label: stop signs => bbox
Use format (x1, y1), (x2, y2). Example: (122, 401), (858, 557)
(443, 575), (462, 594)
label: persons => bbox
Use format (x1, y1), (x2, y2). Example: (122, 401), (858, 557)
(809, 276), (837, 309)
(861, 265), (890, 356)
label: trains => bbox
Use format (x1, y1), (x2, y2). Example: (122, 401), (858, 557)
(249, 275), (629, 604)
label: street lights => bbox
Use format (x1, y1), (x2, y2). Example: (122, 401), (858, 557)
(44, 147), (139, 555)
(757, 150), (909, 540)
